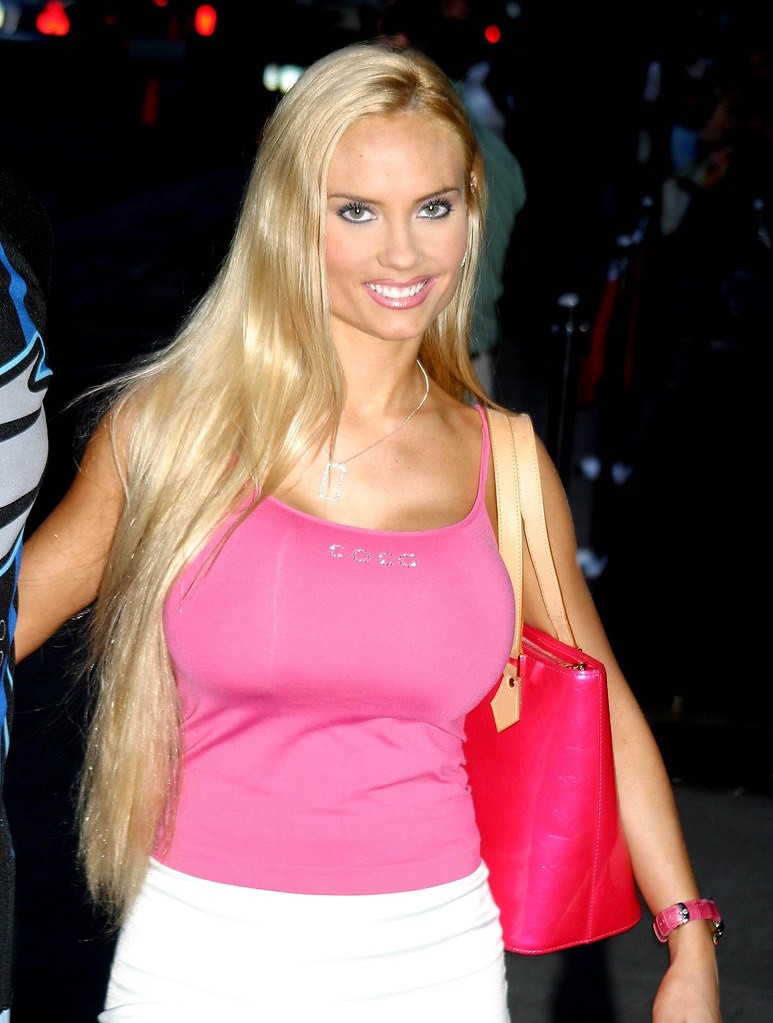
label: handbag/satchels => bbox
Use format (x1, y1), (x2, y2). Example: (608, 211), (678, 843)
(464, 407), (643, 955)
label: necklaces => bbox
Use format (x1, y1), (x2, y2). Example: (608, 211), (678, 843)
(317, 359), (429, 502)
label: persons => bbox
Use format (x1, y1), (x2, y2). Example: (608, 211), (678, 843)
(0, 18), (724, 1023)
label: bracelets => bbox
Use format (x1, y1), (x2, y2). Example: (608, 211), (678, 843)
(653, 897), (723, 948)
